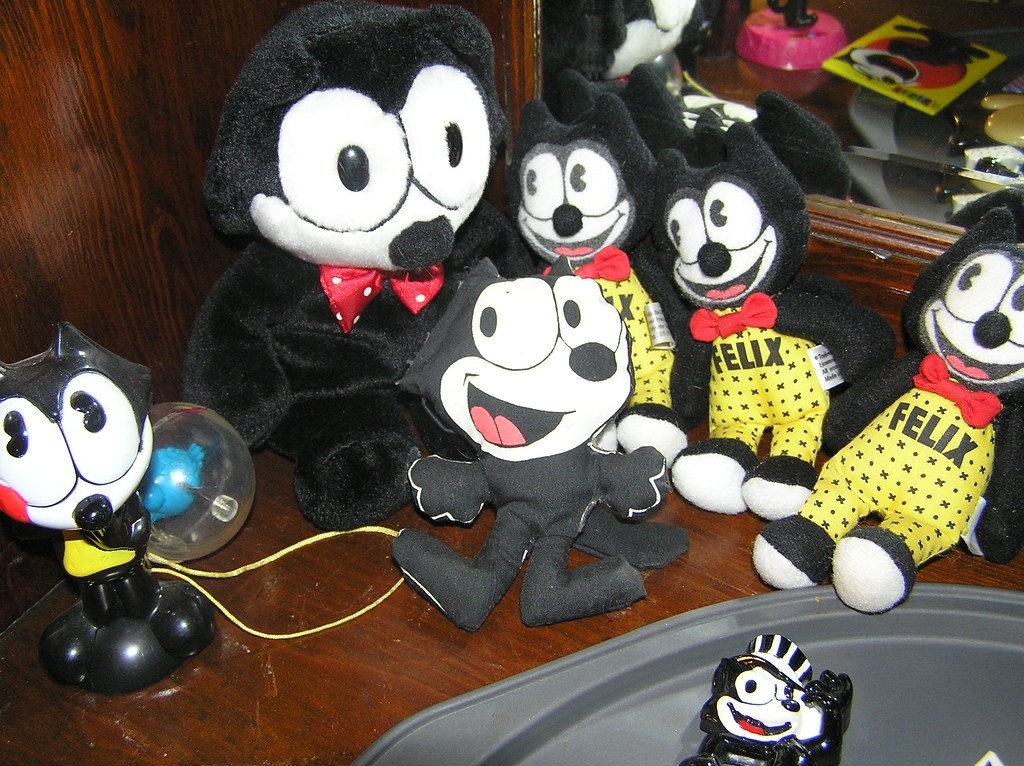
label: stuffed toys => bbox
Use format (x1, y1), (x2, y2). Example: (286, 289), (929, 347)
(501, 94), (697, 467)
(653, 117), (899, 520)
(540, 1), (851, 197)
(754, 203), (1024, 614)
(177, 0), (517, 528)
(399, 255), (690, 627)
(947, 189), (1024, 242)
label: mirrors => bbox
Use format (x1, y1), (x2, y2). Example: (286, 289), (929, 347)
(499, 0), (1024, 269)
